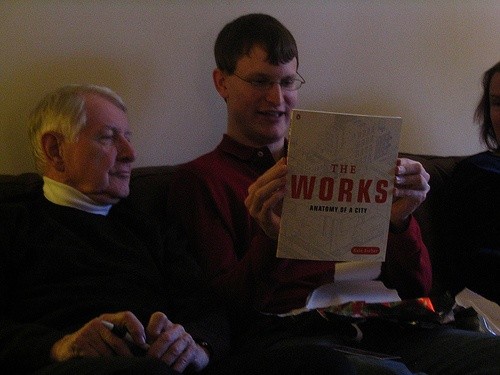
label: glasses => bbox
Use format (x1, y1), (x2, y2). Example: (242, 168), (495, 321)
(231, 72), (306, 91)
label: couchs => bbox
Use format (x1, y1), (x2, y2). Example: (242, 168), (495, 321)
(0, 155), (463, 375)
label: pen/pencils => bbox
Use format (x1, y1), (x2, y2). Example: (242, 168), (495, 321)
(100, 319), (152, 352)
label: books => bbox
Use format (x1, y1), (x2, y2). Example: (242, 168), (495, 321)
(276, 110), (403, 262)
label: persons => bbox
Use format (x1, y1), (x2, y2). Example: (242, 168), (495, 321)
(161, 13), (500, 375)
(430, 62), (500, 307)
(0, 83), (236, 375)
